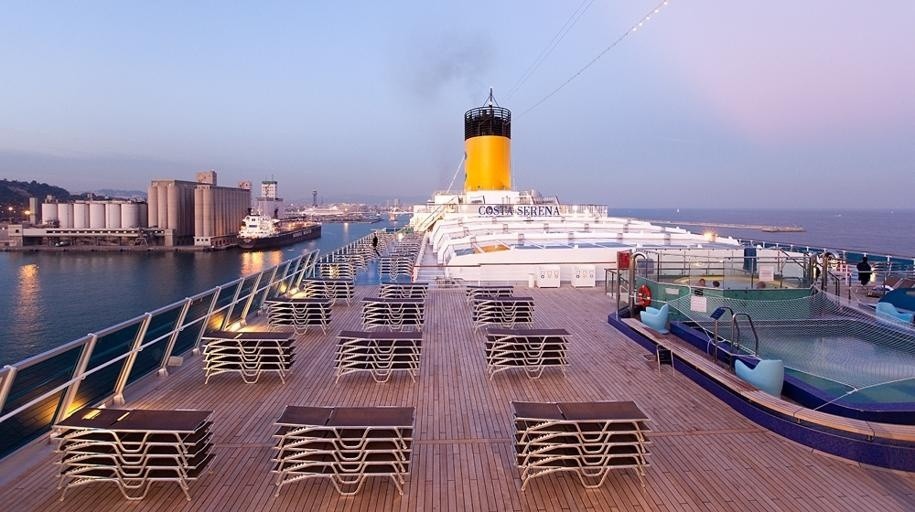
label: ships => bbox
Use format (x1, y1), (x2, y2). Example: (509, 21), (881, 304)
(236, 207), (321, 250)
(0, 85), (915, 510)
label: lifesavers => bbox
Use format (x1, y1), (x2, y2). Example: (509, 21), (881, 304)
(636, 285), (651, 306)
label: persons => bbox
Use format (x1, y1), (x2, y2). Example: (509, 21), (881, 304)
(836, 261), (841, 271)
(693, 278), (722, 298)
(856, 256), (873, 289)
(372, 235), (378, 247)
(816, 266), (820, 281)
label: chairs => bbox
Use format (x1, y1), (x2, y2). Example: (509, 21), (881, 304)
(264, 297), (334, 336)
(466, 284), (514, 309)
(51, 408), (217, 502)
(302, 231), (429, 297)
(269, 404), (415, 498)
(472, 296), (537, 333)
(875, 302), (912, 322)
(484, 328), (573, 380)
(333, 331), (423, 383)
(641, 343), (675, 378)
(511, 401), (652, 493)
(734, 358), (784, 400)
(361, 297), (425, 333)
(201, 331), (296, 385)
(639, 304), (669, 334)
